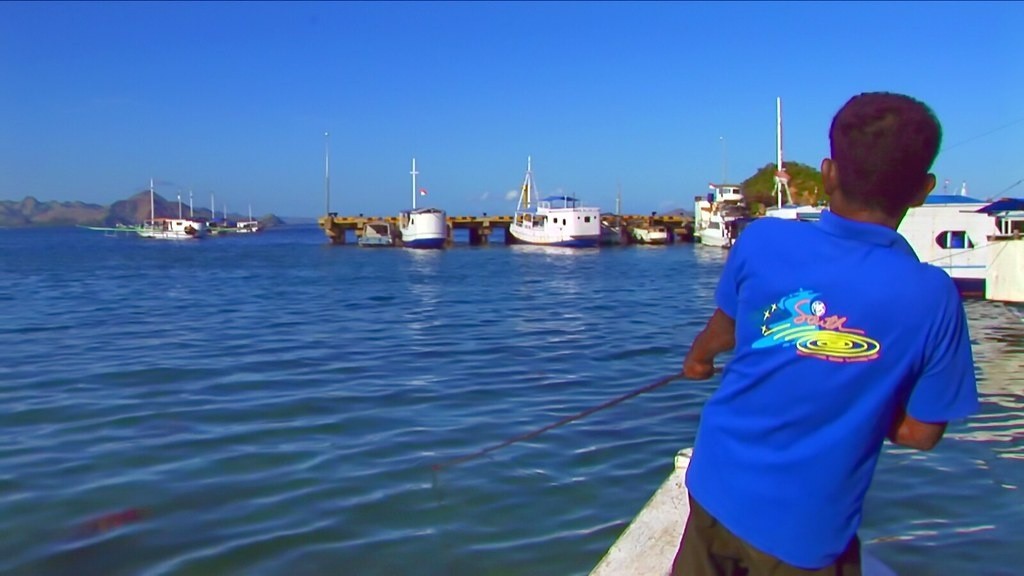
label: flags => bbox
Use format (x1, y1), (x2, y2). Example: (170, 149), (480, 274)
(419, 188), (429, 196)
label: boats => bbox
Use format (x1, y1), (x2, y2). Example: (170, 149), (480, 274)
(693, 184), (748, 247)
(896, 183), (1000, 291)
(510, 156), (602, 250)
(400, 157), (447, 250)
(631, 224), (670, 245)
(358, 222), (393, 248)
(764, 95), (830, 221)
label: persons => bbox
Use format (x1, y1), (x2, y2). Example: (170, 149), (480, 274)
(671, 91), (982, 576)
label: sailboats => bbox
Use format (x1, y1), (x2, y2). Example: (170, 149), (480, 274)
(133, 178), (261, 240)
